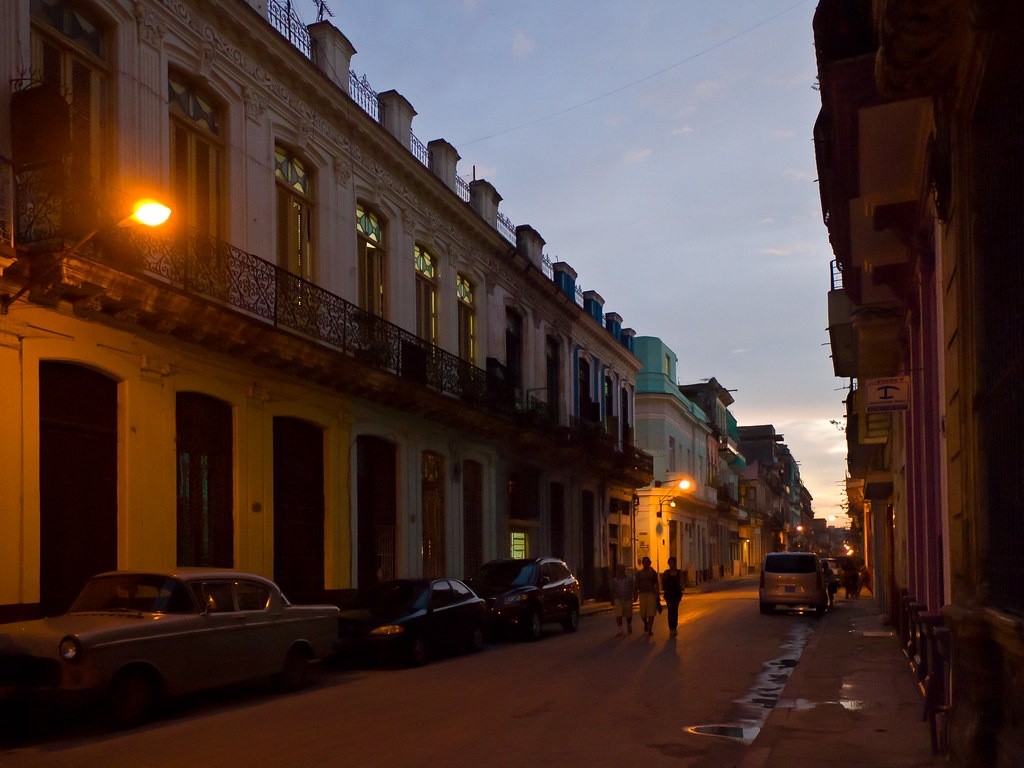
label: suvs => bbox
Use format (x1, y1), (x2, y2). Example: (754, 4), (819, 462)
(463, 556), (581, 644)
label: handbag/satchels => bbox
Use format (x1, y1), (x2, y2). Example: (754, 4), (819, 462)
(657, 597), (664, 615)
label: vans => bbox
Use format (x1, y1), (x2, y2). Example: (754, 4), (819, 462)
(758, 551), (829, 617)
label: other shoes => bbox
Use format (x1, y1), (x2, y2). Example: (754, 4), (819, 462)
(616, 626), (632, 636)
(644, 622), (654, 636)
(671, 629), (677, 636)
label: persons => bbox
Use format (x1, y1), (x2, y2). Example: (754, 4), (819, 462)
(634, 556), (661, 636)
(839, 556), (868, 601)
(662, 557), (685, 638)
(608, 565), (638, 637)
(822, 561), (838, 608)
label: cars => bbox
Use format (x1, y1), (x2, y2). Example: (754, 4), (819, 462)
(1, 566), (342, 730)
(820, 558), (842, 585)
(336, 576), (487, 669)
